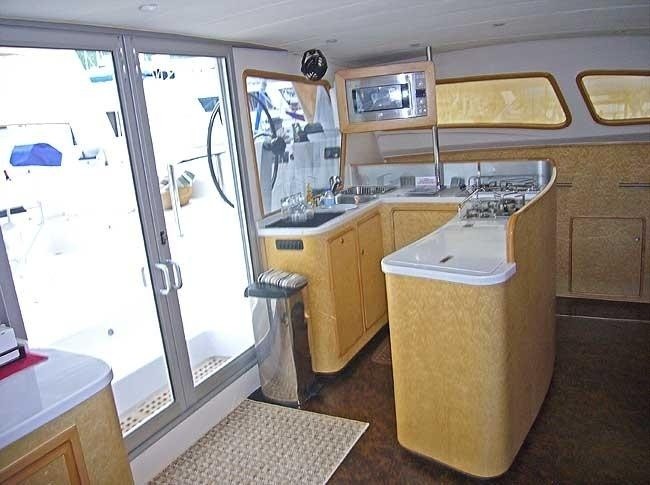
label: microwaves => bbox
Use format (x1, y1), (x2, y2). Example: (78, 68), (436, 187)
(344, 69), (428, 125)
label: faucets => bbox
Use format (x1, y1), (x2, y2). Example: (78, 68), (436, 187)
(330, 176), (342, 193)
(457, 187), (485, 223)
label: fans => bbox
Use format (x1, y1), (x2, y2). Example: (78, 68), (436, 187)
(301, 48), (327, 81)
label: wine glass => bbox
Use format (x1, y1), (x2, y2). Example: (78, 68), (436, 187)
(281, 191), (314, 223)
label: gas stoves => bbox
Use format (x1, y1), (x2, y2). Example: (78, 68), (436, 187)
(459, 192), (535, 220)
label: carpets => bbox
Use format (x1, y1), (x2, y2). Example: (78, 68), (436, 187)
(151, 399), (370, 485)
(117, 356), (231, 436)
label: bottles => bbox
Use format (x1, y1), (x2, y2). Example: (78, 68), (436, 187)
(324, 189), (334, 208)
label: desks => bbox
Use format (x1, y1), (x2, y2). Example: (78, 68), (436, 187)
(159, 151), (224, 237)
(1, 348), (135, 485)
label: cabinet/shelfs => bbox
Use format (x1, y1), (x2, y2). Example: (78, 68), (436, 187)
(571, 216), (640, 298)
(327, 210), (384, 357)
(390, 207), (457, 251)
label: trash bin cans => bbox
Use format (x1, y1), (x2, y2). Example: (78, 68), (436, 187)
(243, 281), (319, 410)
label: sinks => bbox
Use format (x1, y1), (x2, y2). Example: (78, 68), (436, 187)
(334, 194), (378, 205)
(340, 183), (397, 195)
(388, 223), (507, 274)
